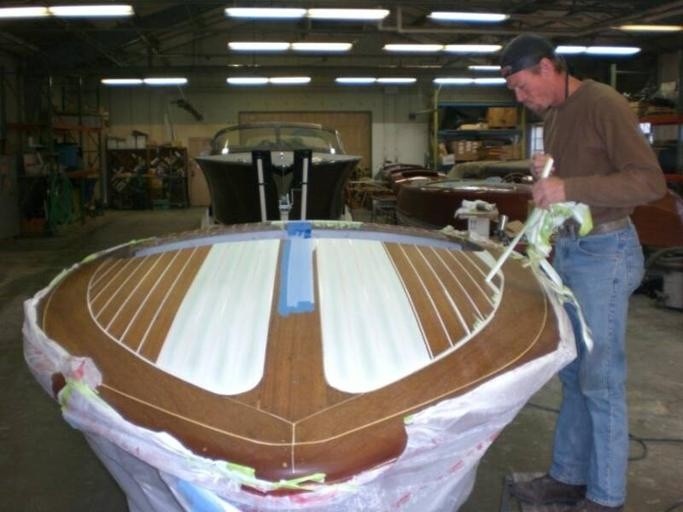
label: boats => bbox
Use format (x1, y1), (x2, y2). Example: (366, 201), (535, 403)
(194, 120), (365, 225)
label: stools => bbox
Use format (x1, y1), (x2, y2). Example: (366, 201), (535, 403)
(371, 196), (399, 224)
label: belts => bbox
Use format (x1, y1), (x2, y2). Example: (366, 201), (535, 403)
(575, 218), (628, 234)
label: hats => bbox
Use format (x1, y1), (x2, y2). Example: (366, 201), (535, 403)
(498, 31), (556, 77)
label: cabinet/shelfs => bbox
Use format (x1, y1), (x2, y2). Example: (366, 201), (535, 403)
(429, 88), (683, 173)
(19, 69), (189, 219)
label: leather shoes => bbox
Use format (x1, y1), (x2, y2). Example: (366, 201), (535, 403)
(508, 475), (586, 503)
(561, 495), (623, 511)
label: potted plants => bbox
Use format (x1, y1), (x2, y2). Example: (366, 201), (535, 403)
(19, 164), (79, 236)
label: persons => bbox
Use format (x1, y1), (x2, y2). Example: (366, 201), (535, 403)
(497, 31), (667, 510)
